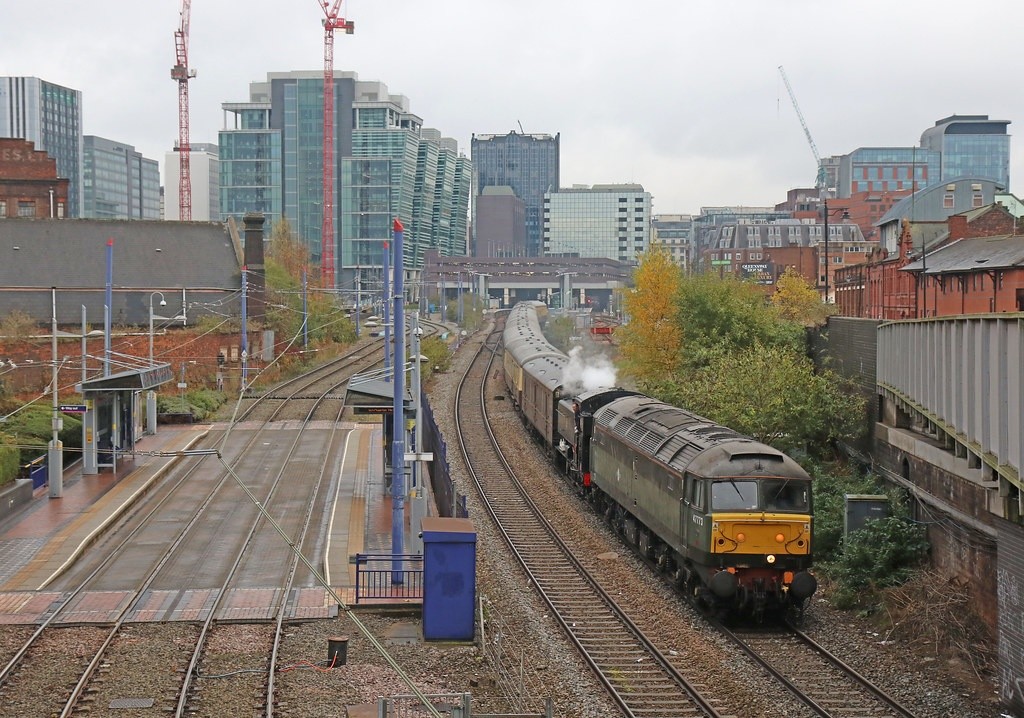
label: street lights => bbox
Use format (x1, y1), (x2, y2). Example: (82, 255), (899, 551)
(146, 291), (167, 434)
(410, 311), (427, 558)
(823, 199), (853, 304)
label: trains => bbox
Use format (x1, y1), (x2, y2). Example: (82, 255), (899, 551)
(502, 300), (818, 631)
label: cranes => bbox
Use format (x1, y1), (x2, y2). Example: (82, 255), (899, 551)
(170, 0), (197, 221)
(776, 65), (820, 169)
(319, 0), (354, 292)
